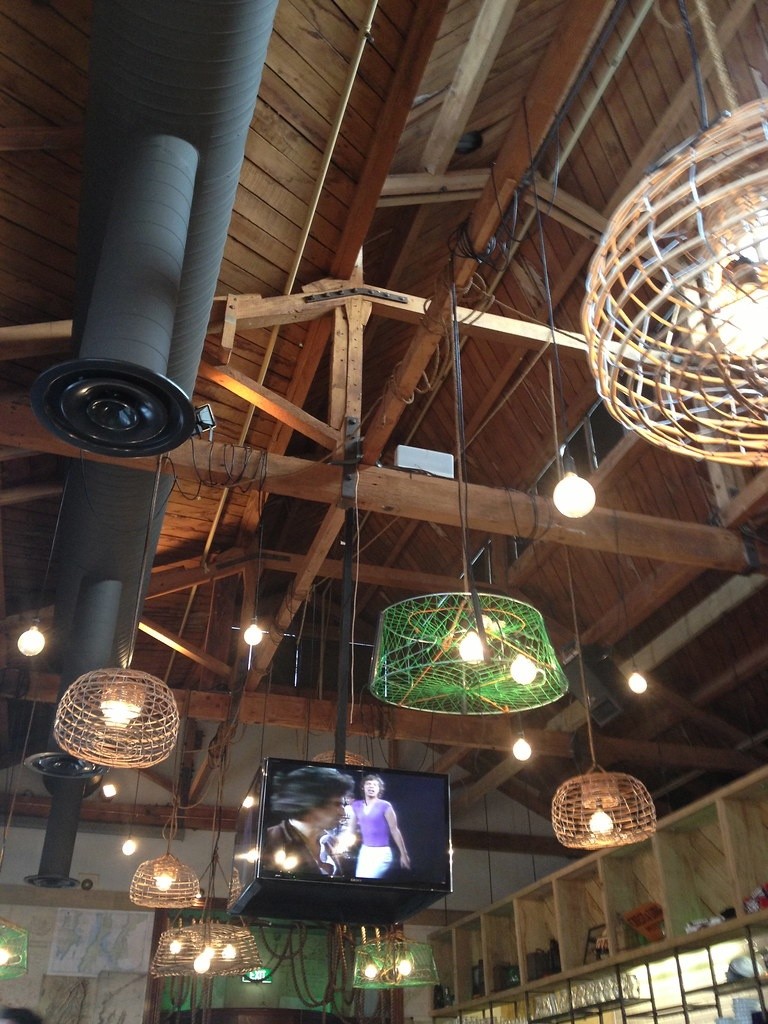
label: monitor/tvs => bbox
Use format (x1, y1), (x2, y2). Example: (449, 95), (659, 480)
(256, 756), (453, 895)
(226, 760), (265, 913)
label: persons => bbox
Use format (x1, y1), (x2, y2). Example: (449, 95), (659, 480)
(262, 767), (410, 877)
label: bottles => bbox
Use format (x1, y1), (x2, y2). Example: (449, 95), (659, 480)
(471, 959), (484, 995)
(444, 988), (452, 1007)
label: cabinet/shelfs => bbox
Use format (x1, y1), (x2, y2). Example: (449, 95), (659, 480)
(430, 767), (768, 1024)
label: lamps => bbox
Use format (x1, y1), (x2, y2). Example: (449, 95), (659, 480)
(51, 2), (768, 988)
